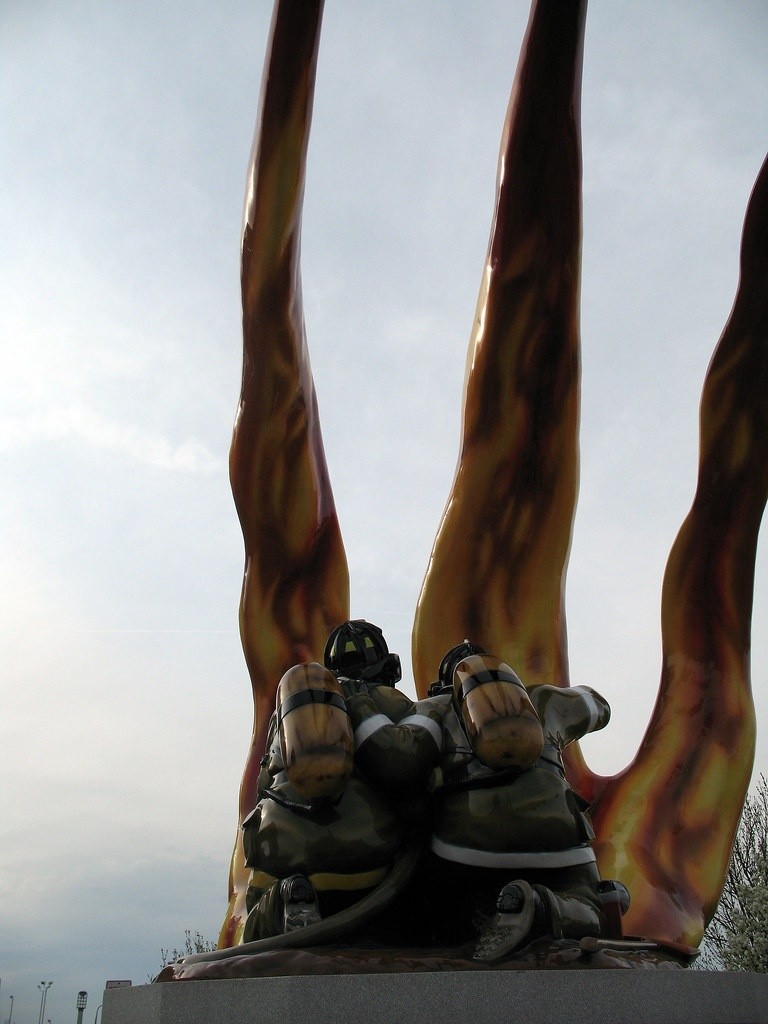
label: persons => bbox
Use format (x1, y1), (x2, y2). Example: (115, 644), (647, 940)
(340, 639), (628, 962)
(240, 620), (431, 948)
